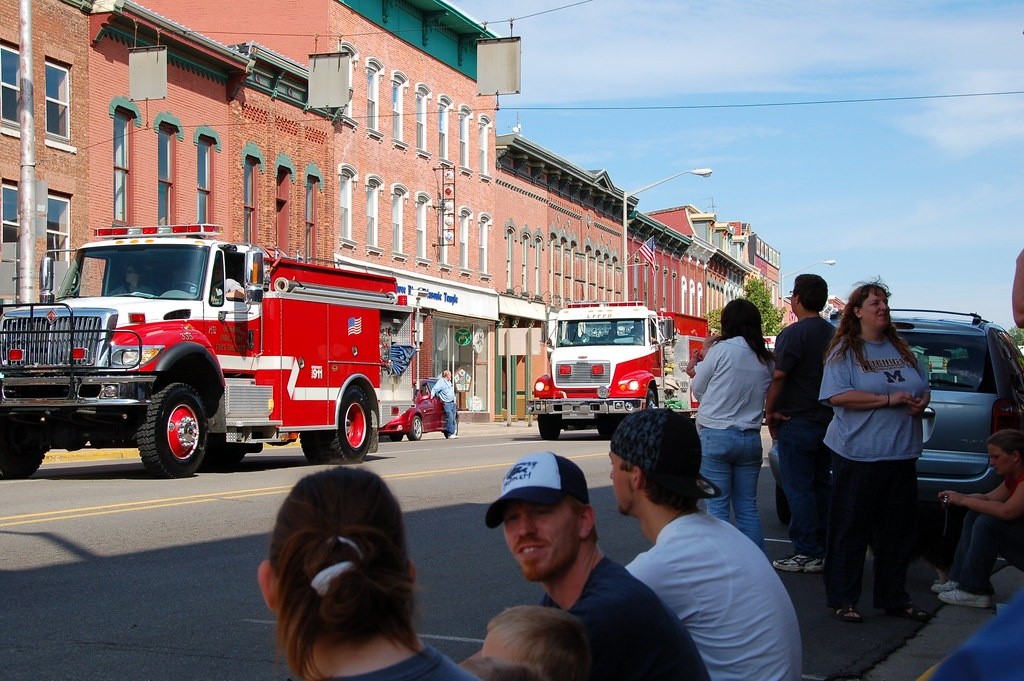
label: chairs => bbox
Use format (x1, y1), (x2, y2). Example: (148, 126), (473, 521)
(947, 357), (976, 384)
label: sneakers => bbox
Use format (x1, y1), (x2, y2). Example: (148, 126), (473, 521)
(938, 586), (990, 608)
(773, 552), (826, 572)
(932, 579), (957, 594)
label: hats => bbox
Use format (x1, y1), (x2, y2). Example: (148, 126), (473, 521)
(485, 451), (589, 528)
(612, 407), (722, 498)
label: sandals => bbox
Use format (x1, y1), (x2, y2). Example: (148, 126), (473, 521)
(886, 601), (928, 620)
(830, 605), (861, 623)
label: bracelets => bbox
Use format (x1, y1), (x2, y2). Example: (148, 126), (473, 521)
(888, 394), (890, 406)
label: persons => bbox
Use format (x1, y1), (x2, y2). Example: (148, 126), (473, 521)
(431, 370), (459, 438)
(632, 320), (659, 344)
(210, 256), (244, 299)
(257, 465), (484, 681)
(485, 451), (713, 681)
(765, 273), (839, 572)
(608, 408), (803, 681)
(103, 262), (154, 297)
(931, 428), (1024, 607)
(817, 276), (932, 620)
(461, 605), (595, 681)
(688, 299), (775, 573)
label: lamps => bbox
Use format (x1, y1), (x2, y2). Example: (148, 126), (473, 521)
(504, 285), (572, 308)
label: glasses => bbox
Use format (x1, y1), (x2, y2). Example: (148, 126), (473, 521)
(790, 291), (795, 295)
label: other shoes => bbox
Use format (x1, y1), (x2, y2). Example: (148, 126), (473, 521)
(449, 433), (459, 439)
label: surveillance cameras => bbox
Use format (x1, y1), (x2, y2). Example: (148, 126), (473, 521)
(411, 290), (429, 298)
(711, 328), (719, 333)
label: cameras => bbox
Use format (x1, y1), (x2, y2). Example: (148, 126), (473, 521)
(942, 494), (951, 504)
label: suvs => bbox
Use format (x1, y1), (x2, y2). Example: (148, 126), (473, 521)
(827, 304), (1023, 558)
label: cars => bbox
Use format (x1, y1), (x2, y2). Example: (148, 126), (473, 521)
(377, 378), (460, 442)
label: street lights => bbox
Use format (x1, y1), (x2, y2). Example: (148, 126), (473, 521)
(620, 167), (714, 304)
(781, 259), (837, 326)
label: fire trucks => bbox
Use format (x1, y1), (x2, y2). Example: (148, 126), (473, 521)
(523, 299), (719, 443)
(0, 223), (422, 481)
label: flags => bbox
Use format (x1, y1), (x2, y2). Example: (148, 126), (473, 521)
(639, 237), (656, 275)
